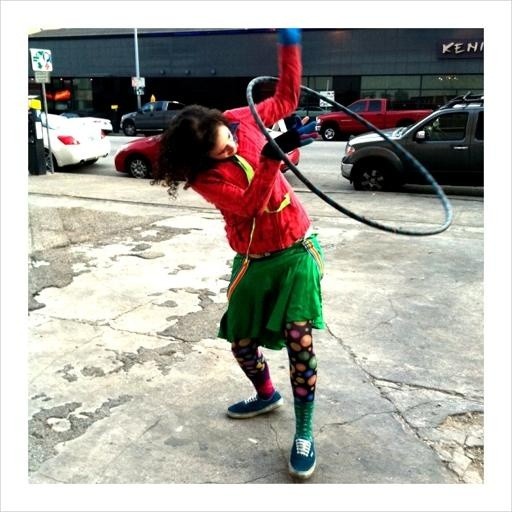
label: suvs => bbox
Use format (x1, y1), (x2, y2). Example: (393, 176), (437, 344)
(339, 94), (483, 200)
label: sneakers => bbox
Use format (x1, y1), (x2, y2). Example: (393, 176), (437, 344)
(288, 434), (317, 480)
(227, 389), (284, 419)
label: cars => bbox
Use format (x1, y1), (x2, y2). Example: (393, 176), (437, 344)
(31, 99), (313, 178)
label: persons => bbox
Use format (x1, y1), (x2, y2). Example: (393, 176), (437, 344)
(147, 29), (326, 479)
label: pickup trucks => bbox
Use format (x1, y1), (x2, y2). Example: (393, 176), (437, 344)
(316, 97), (435, 141)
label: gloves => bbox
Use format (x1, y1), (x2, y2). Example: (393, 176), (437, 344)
(276, 28), (302, 44)
(261, 114), (320, 159)
